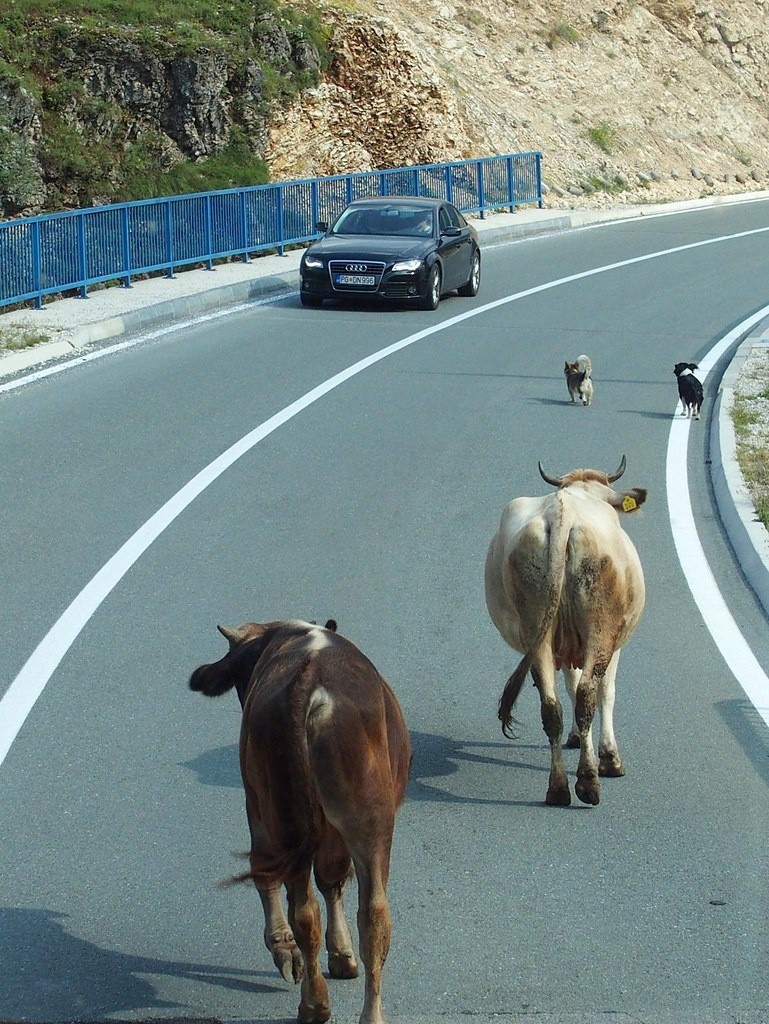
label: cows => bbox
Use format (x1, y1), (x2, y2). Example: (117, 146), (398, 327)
(486, 454), (648, 806)
(190, 618), (414, 1024)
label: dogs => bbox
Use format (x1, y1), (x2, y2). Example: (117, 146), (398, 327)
(564, 354), (593, 406)
(672, 362), (705, 420)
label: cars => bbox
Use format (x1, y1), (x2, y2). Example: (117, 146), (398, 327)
(299, 195), (482, 311)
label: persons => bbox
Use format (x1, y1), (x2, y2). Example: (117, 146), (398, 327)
(412, 212), (432, 234)
